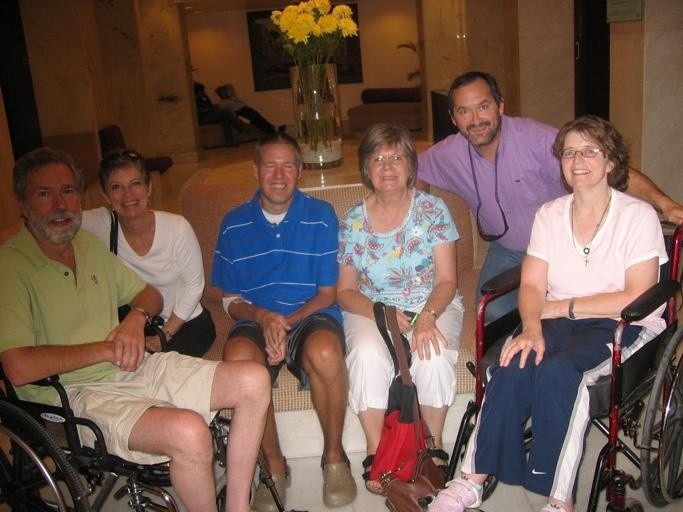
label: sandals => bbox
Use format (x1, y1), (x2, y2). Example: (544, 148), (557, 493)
(361, 454), (386, 495)
(253, 457), (292, 512)
(320, 450), (357, 509)
(426, 448), (450, 484)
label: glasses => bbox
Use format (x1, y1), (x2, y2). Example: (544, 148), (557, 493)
(100, 150), (144, 165)
(558, 147), (606, 158)
(367, 154), (409, 164)
(477, 202), (510, 242)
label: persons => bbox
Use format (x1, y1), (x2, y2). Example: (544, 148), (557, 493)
(1, 148), (273, 512)
(79, 152), (216, 354)
(195, 84), (251, 148)
(337, 123), (466, 495)
(427, 114), (669, 512)
(214, 83), (286, 137)
(210, 133), (356, 512)
(416, 71), (683, 501)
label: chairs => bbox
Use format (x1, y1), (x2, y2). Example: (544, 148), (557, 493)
(100, 123), (174, 205)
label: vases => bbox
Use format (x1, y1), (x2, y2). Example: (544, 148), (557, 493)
(282, 63), (347, 172)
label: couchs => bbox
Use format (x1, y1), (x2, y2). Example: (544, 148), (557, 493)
(78, 165), (526, 462)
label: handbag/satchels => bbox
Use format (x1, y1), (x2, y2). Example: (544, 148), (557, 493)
(369, 302), (436, 497)
(378, 447), (449, 512)
(109, 211), (165, 337)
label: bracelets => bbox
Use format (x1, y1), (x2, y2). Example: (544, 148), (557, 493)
(569, 297), (575, 320)
(162, 326), (175, 338)
(422, 308), (439, 319)
(130, 305), (155, 327)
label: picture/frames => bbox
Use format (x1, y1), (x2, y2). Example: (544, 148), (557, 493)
(245, 2), (365, 96)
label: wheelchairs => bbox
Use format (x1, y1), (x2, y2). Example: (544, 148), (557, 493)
(0, 315), (290, 512)
(440, 220), (682, 512)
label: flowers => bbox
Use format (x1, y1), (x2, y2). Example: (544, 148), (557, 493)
(269, 0), (361, 152)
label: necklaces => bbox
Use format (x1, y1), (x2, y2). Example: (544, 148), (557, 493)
(571, 196), (610, 269)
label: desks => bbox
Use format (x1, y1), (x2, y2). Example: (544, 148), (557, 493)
(429, 86), (461, 146)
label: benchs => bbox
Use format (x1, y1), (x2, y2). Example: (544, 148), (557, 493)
(345, 81), (423, 136)
(193, 113), (265, 148)
